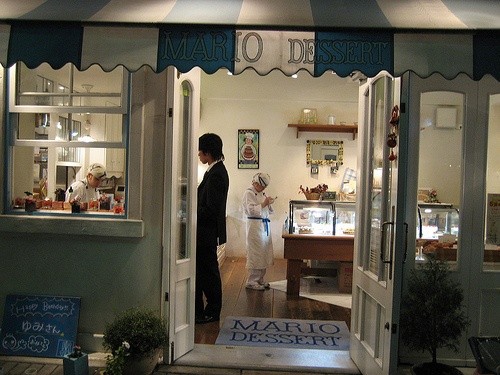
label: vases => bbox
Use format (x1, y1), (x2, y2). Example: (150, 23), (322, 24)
(63, 351), (89, 375)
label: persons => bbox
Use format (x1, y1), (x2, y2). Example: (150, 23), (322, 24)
(65, 163), (106, 203)
(195, 134), (229, 324)
(244, 172), (276, 291)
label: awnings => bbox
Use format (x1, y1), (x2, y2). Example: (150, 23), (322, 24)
(0, 0), (500, 80)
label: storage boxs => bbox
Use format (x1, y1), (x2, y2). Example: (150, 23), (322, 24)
(336, 261), (352, 293)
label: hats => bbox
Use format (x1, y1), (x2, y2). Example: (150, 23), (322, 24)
(89, 164), (107, 180)
(252, 172), (271, 186)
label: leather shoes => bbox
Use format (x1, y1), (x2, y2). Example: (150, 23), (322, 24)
(197, 312), (219, 323)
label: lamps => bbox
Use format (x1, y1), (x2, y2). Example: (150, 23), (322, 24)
(81, 84), (93, 131)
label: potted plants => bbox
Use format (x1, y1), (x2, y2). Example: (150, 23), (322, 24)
(102, 305), (171, 375)
(397, 254), (472, 375)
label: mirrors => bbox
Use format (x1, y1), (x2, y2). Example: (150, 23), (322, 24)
(306, 139), (343, 166)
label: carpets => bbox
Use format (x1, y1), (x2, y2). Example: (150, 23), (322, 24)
(215, 316), (350, 350)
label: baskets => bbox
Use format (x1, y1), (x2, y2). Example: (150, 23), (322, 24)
(305, 192), (320, 200)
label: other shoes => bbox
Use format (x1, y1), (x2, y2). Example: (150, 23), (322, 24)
(246, 282), (265, 290)
(258, 278), (269, 288)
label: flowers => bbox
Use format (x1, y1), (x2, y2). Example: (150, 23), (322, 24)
(68, 345), (83, 358)
